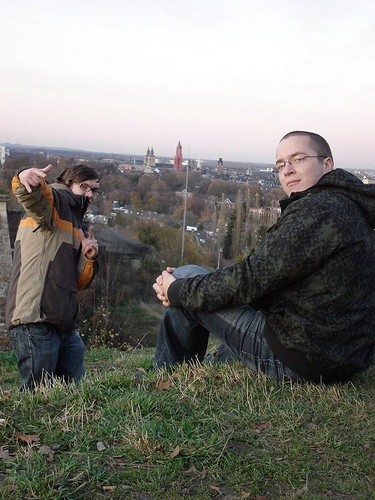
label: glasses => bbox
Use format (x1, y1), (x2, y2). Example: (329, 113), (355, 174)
(73, 181), (102, 196)
(273, 155), (327, 173)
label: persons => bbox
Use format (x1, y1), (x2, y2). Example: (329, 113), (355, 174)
(6, 163), (103, 394)
(151, 131), (375, 387)
